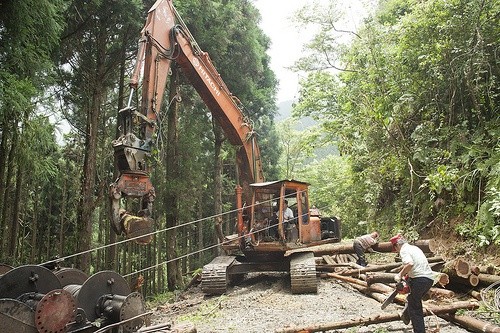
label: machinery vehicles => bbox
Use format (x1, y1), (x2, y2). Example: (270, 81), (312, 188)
(106, 0), (342, 297)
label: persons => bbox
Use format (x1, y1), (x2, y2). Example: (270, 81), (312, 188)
(275, 199), (295, 239)
(353, 232), (379, 267)
(390, 233), (435, 333)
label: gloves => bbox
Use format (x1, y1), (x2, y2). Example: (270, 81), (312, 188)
(394, 274), (401, 283)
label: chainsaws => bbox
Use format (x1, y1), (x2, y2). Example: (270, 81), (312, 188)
(380, 274), (411, 311)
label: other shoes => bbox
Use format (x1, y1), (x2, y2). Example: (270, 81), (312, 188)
(406, 330), (414, 333)
(398, 310), (410, 325)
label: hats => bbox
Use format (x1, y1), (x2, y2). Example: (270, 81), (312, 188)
(390, 233), (401, 253)
